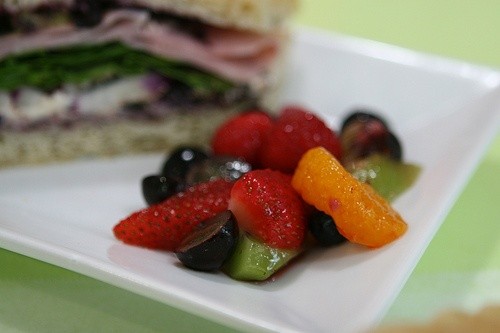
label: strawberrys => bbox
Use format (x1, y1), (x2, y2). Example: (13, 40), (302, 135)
(113, 179), (231, 248)
(258, 108), (342, 172)
(210, 108), (276, 167)
(228, 168), (309, 249)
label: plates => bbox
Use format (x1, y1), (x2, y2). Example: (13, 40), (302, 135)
(0, 24), (500, 333)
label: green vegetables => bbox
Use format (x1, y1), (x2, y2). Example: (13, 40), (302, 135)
(0, 39), (240, 97)
